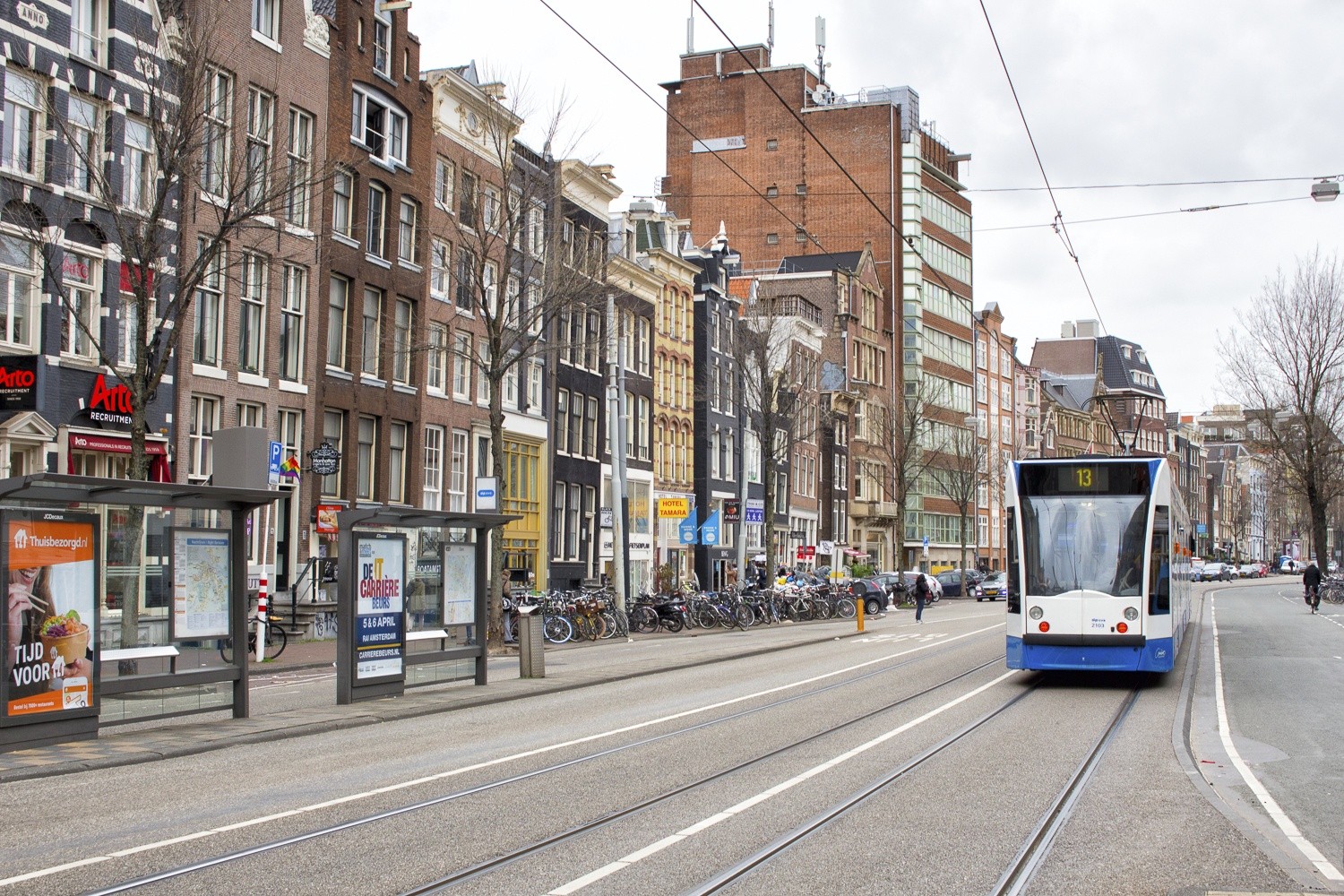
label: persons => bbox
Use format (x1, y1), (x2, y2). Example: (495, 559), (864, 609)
(786, 571), (795, 582)
(8, 565), (93, 701)
(320, 514), (337, 527)
(872, 565), (879, 576)
(980, 562), (989, 575)
(1288, 559), (1295, 575)
(695, 573), (699, 592)
(1303, 561), (1321, 610)
(1240, 559), (1244, 564)
(406, 572), (427, 632)
(727, 564), (737, 584)
(1273, 561), (1279, 574)
(752, 561), (766, 590)
(776, 568), (787, 580)
(915, 574), (930, 622)
(501, 569), (516, 644)
(1250, 559), (1254, 564)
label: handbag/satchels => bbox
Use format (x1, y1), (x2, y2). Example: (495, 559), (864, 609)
(502, 595), (511, 611)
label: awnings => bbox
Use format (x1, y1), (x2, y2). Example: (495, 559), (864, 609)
(844, 550), (871, 558)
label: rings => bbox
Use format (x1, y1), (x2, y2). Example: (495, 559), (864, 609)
(9, 608), (11, 610)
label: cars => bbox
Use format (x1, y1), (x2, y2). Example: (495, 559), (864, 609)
(1299, 561), (1309, 574)
(1281, 560), (1300, 575)
(839, 577), (889, 615)
(942, 569), (984, 585)
(1252, 564), (1270, 578)
(975, 573), (1008, 602)
(1238, 565), (1254, 578)
(868, 571), (943, 606)
(935, 572), (977, 598)
(1199, 562), (1230, 583)
(1228, 565), (1241, 580)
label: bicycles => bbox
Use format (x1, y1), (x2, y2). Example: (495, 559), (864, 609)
(504, 571), (859, 643)
(1318, 571), (1344, 603)
(1307, 585), (1318, 613)
(219, 606), (322, 664)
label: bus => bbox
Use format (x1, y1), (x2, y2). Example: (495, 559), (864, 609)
(1006, 395), (1196, 676)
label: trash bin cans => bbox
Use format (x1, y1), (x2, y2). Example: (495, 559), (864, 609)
(518, 606), (546, 679)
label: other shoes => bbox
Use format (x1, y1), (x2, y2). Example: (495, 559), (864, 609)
(917, 620), (923, 623)
